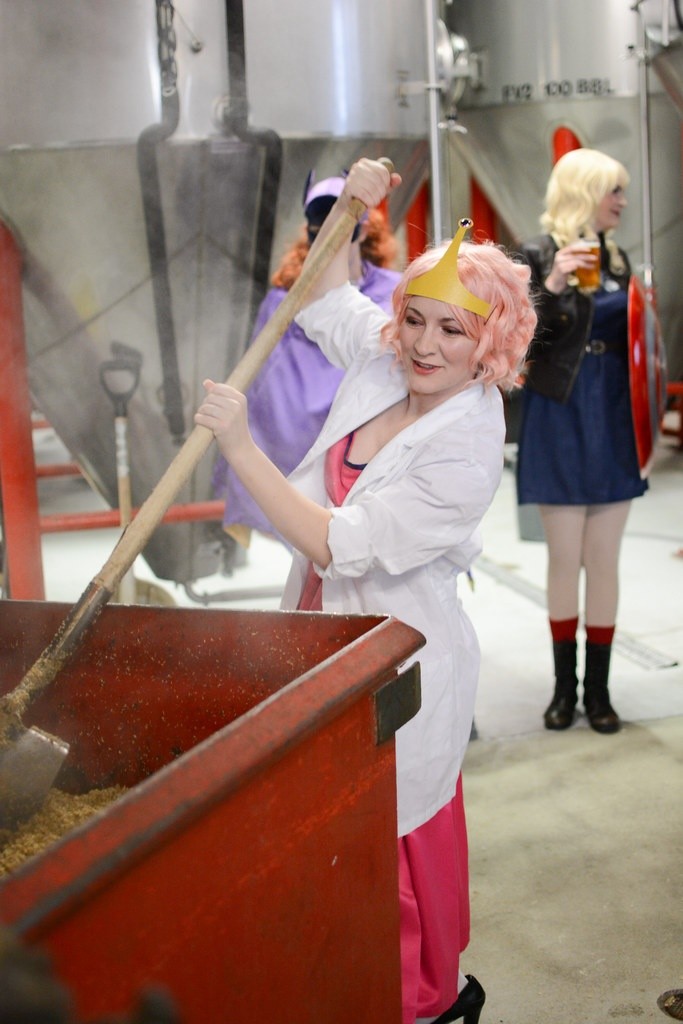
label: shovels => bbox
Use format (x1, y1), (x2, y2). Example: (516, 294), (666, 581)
(96, 361), (176, 606)
(0, 154), (396, 855)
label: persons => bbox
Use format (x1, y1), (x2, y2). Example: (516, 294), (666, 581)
(196, 157), (538, 1023)
(224, 168), (400, 611)
(520, 148), (633, 734)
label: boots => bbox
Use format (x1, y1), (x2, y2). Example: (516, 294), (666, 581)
(544, 639), (577, 729)
(584, 641), (619, 734)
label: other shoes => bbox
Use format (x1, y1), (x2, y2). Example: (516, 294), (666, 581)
(657, 989), (683, 1021)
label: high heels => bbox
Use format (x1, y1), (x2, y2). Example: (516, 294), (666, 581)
(432, 974), (485, 1024)
